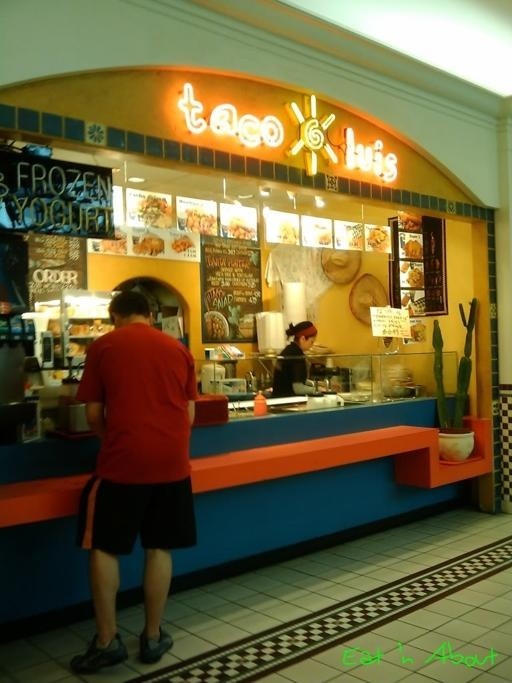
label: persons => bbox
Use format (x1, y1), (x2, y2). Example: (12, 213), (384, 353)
(272, 321), (331, 398)
(70, 290), (198, 675)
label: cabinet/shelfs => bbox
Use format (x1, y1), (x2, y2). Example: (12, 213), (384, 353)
(32, 290), (121, 370)
(38, 352), (460, 444)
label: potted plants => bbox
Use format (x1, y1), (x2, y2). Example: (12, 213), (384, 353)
(432, 298), (476, 462)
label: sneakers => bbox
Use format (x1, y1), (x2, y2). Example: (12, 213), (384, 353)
(68, 631), (127, 674)
(138, 625), (173, 663)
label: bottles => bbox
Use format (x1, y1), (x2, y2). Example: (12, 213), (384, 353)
(259, 373), (265, 391)
(265, 370), (271, 389)
